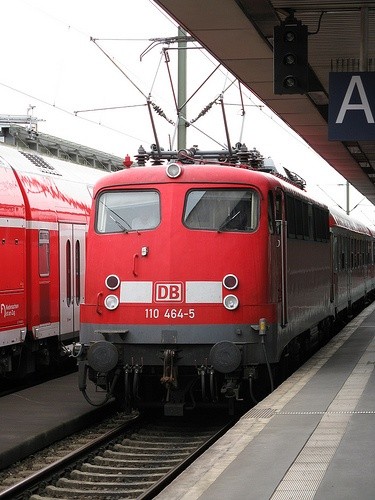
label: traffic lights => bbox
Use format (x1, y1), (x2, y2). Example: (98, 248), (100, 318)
(276, 17), (312, 98)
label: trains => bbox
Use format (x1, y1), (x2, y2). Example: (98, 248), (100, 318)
(0, 115), (137, 393)
(77, 142), (374, 411)
